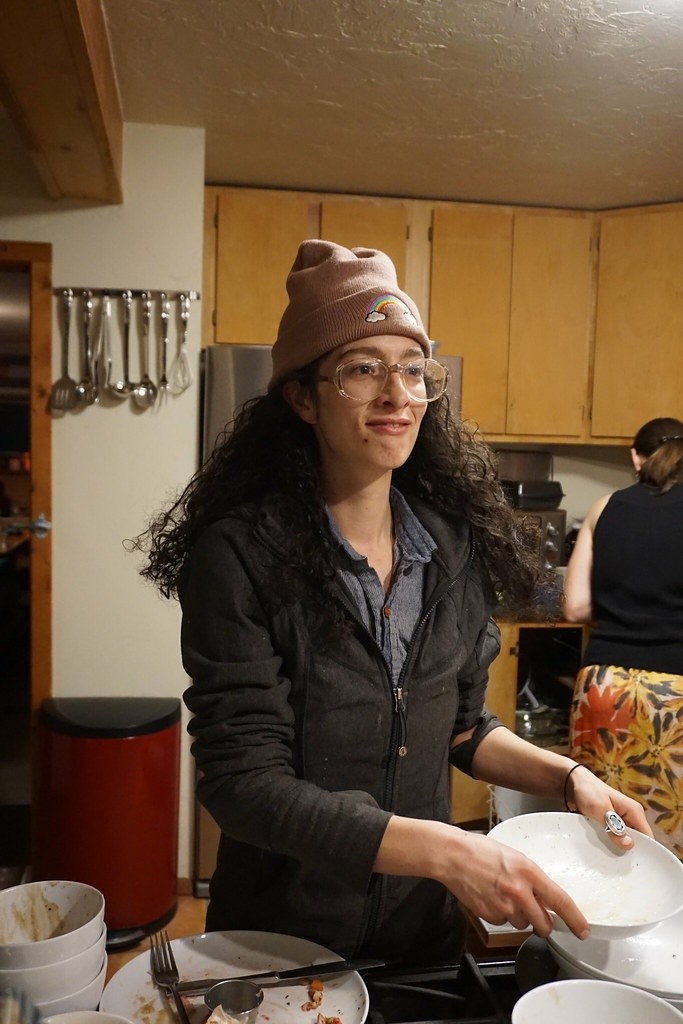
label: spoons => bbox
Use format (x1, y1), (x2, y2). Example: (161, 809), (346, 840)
(133, 291), (157, 410)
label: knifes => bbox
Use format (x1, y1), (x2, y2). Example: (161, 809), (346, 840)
(165, 956), (386, 999)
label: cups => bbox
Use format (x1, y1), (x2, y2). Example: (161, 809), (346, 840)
(204, 979), (264, 1024)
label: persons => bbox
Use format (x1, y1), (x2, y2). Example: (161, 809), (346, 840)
(584, 418), (683, 682)
(181, 240), (655, 969)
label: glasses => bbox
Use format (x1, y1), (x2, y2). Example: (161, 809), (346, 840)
(298, 357), (451, 403)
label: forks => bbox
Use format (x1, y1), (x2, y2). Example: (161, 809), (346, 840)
(149, 929), (191, 1024)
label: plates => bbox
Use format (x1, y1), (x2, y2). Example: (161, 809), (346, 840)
(486, 812), (683, 940)
(98, 930), (370, 1024)
(544, 907), (683, 1014)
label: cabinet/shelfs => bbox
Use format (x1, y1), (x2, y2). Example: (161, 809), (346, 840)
(427, 197), (593, 445)
(589, 201), (683, 446)
(212, 185), (410, 348)
(446, 615), (596, 833)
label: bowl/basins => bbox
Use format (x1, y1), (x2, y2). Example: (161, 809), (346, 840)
(511, 978), (683, 1024)
(0, 880), (134, 1024)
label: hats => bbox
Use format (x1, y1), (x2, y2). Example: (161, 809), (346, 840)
(267, 239), (432, 390)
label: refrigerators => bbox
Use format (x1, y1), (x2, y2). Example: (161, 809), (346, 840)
(194, 341), (464, 899)
